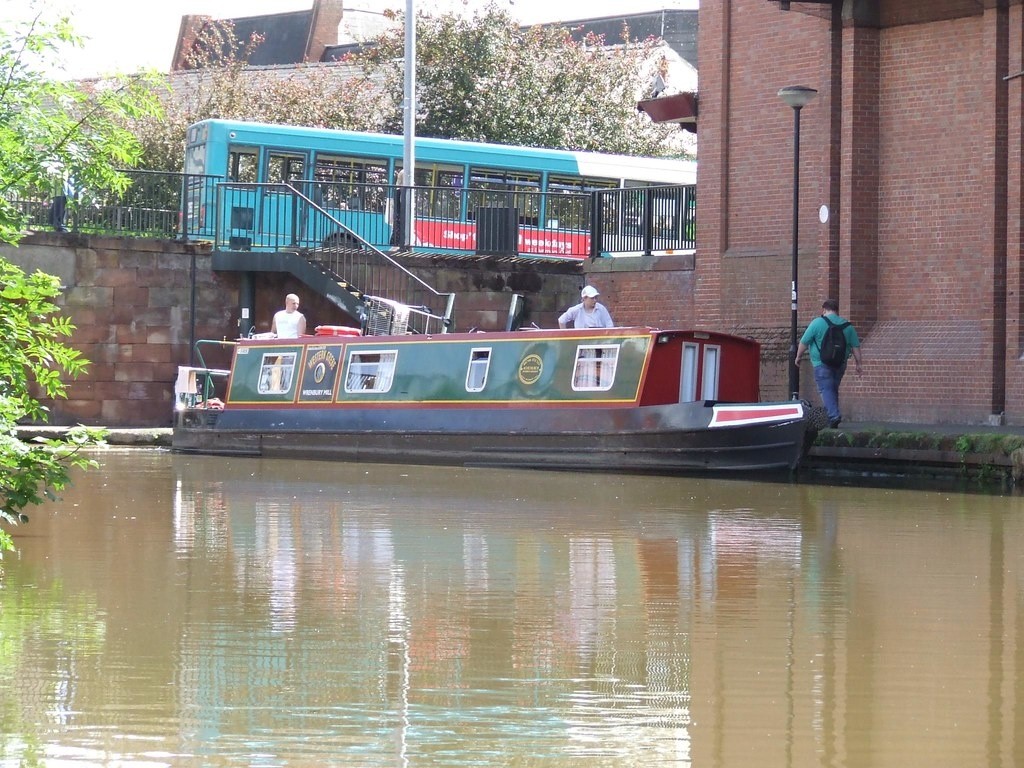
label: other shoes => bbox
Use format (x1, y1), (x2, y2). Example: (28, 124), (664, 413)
(830, 417), (841, 428)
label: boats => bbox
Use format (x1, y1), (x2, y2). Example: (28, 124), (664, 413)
(172, 294), (829, 477)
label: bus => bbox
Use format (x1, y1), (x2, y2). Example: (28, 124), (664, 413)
(178, 119), (698, 261)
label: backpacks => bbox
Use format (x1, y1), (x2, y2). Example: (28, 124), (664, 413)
(814, 315), (853, 368)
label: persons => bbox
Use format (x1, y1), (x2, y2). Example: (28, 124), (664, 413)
(795, 300), (863, 428)
(271, 293), (306, 338)
(558, 286), (614, 329)
(46, 162), (69, 232)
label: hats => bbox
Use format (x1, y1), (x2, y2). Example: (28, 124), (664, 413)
(581, 286), (600, 298)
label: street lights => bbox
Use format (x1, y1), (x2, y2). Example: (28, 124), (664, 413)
(777, 85), (818, 403)
